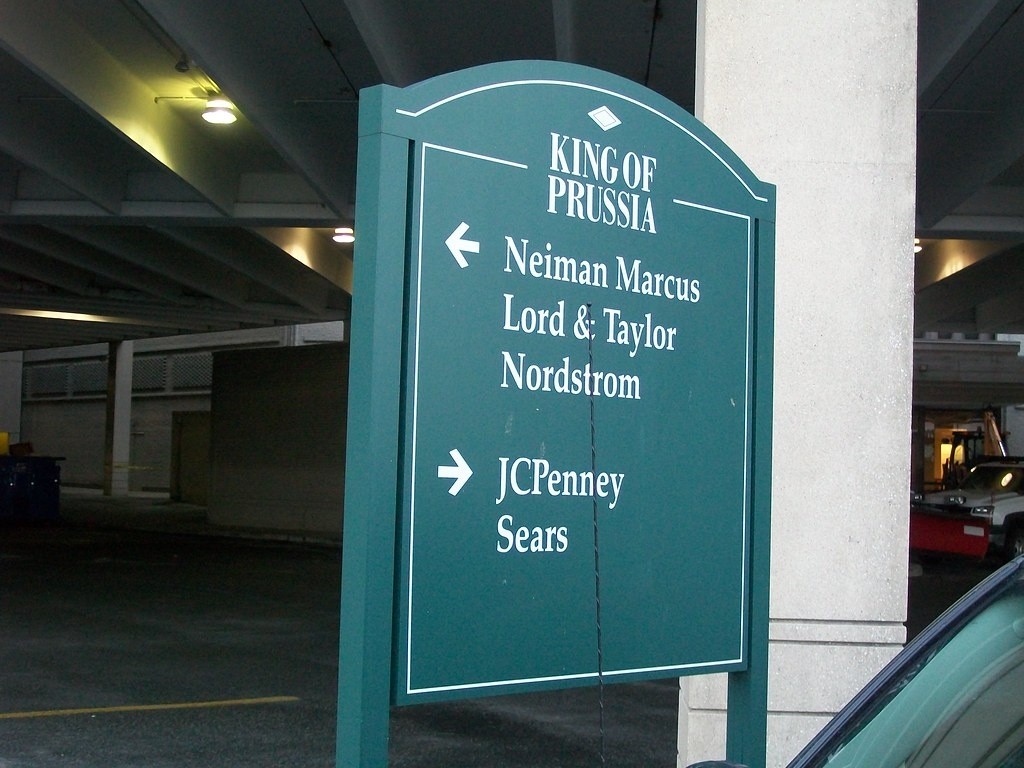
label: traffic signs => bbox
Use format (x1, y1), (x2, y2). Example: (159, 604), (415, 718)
(331, 57), (779, 768)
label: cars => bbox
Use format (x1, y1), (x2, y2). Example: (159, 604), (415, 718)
(678, 550), (1024, 768)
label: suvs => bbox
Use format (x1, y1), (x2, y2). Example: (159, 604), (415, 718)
(909, 456), (1024, 569)
